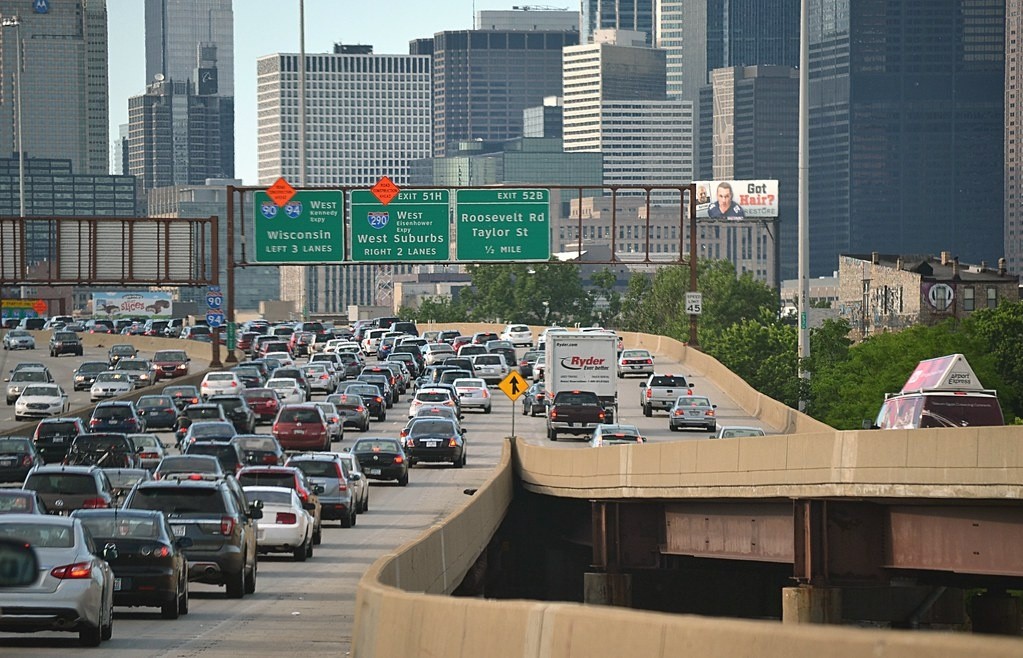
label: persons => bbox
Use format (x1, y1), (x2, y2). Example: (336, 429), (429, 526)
(707, 182), (744, 218)
(696, 186), (710, 205)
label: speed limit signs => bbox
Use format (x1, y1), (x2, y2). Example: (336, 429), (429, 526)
(685, 291), (702, 317)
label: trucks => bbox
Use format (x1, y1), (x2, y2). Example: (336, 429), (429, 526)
(541, 331), (618, 426)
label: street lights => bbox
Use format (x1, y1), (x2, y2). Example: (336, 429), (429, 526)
(1, 9), (27, 217)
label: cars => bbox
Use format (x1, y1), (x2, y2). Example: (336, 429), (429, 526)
(10, 362), (51, 379)
(160, 470), (264, 572)
(4, 367), (55, 405)
(15, 383), (71, 422)
(2, 329), (36, 351)
(639, 374), (696, 417)
(0, 312), (625, 516)
(278, 449), (361, 528)
(583, 424), (647, 448)
(48, 330), (84, 358)
(0, 512), (115, 647)
(241, 486), (316, 561)
(619, 349), (656, 380)
(543, 390), (607, 441)
(708, 426), (766, 439)
(68, 508), (195, 620)
(233, 464), (325, 544)
(668, 395), (718, 433)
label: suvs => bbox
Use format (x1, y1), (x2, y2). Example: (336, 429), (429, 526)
(119, 476), (265, 599)
